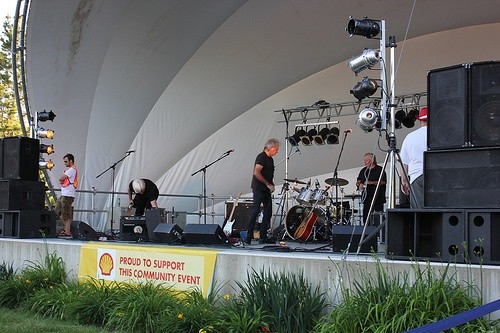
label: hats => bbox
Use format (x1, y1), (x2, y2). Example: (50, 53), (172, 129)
(416, 107), (428, 121)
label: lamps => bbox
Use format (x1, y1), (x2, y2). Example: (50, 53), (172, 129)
(299, 125), (317, 145)
(35, 127), (55, 139)
(285, 126), (306, 147)
(312, 124), (330, 145)
(327, 124), (340, 144)
(39, 158), (55, 171)
(374, 95), (421, 131)
(40, 143), (54, 155)
(37, 110), (56, 122)
(345, 15), (382, 39)
(354, 107), (382, 133)
(350, 76), (383, 101)
(348, 47), (382, 76)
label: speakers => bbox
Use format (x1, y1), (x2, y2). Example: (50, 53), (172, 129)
(332, 225), (377, 252)
(70, 221), (99, 241)
(182, 223), (228, 245)
(145, 209), (167, 241)
(224, 200), (272, 231)
(385, 61), (500, 266)
(153, 223), (184, 245)
(0, 136), (57, 238)
(120, 216), (146, 241)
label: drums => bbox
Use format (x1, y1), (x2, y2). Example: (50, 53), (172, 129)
(295, 187), (313, 205)
(312, 197), (334, 216)
(309, 189), (328, 205)
(284, 205), (326, 242)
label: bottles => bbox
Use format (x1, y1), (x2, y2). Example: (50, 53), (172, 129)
(117, 198), (120, 207)
(376, 237), (380, 251)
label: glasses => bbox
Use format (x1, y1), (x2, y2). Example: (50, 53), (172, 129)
(64, 161), (68, 163)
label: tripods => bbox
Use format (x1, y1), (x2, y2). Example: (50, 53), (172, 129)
(309, 205), (335, 241)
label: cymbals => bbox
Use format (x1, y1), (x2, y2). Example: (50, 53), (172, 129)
(294, 188), (300, 193)
(344, 195), (362, 198)
(284, 179), (307, 184)
(324, 178), (349, 186)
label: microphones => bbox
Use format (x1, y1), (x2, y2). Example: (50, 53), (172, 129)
(126, 150), (136, 153)
(316, 180), (320, 188)
(224, 149), (234, 154)
(296, 147), (301, 154)
(344, 129), (352, 133)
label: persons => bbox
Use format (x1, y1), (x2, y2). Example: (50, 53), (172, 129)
(397, 107), (427, 209)
(128, 178), (158, 216)
(56, 154), (80, 239)
(355, 153), (387, 236)
(245, 138), (280, 244)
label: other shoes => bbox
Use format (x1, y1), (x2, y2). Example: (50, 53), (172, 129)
(259, 239), (275, 244)
(60, 227), (65, 234)
(244, 237), (251, 244)
(58, 234), (73, 239)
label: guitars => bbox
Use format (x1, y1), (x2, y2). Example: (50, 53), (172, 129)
(294, 185), (330, 242)
(221, 192), (243, 238)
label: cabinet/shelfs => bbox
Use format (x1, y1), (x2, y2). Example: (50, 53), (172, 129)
(107, 207), (186, 229)
